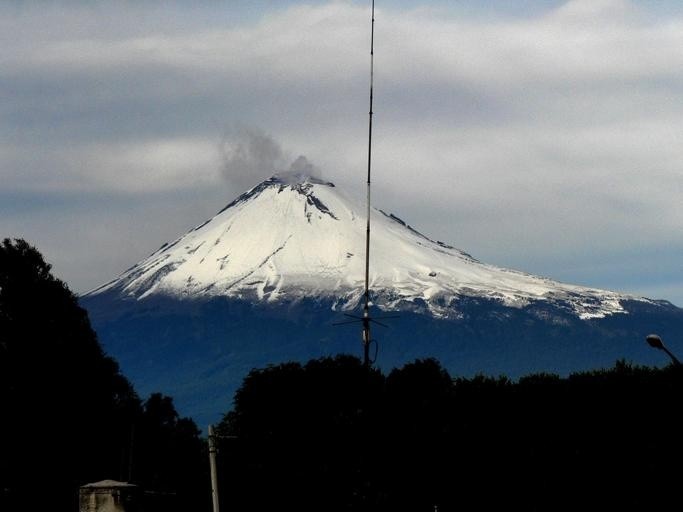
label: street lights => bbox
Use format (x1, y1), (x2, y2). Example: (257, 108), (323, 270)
(646, 332), (681, 386)
